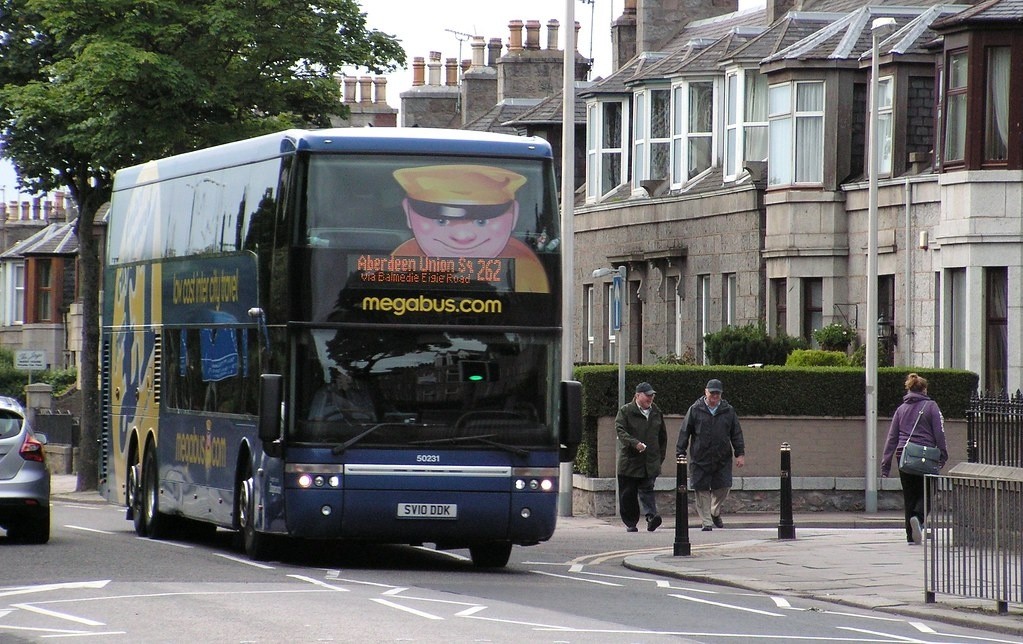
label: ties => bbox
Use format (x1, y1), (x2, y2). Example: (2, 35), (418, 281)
(342, 392), (353, 419)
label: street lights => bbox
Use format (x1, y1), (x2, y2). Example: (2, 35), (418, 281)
(864, 17), (899, 516)
(590, 264), (628, 516)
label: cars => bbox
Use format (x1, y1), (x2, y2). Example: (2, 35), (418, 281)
(0, 396), (48, 546)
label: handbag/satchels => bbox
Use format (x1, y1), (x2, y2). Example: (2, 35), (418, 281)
(899, 442), (940, 474)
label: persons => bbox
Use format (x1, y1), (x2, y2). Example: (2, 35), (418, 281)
(308, 361), (379, 424)
(163, 348), (278, 414)
(675, 378), (746, 531)
(480, 362), (542, 412)
(614, 381), (667, 532)
(880, 373), (948, 544)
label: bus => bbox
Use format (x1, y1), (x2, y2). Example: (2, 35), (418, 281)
(101, 127), (560, 576)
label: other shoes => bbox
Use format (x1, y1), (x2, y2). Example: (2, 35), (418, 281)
(711, 514), (723, 528)
(646, 514), (662, 531)
(701, 526), (712, 531)
(626, 526), (638, 532)
(910, 516), (923, 544)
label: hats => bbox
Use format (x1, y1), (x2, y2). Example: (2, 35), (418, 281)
(706, 379), (723, 393)
(636, 382), (657, 396)
